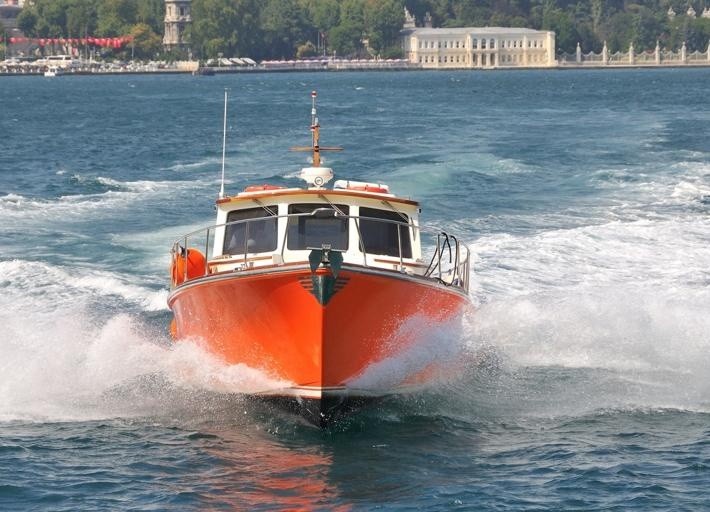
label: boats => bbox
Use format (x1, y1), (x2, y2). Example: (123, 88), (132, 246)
(160, 85), (475, 426)
